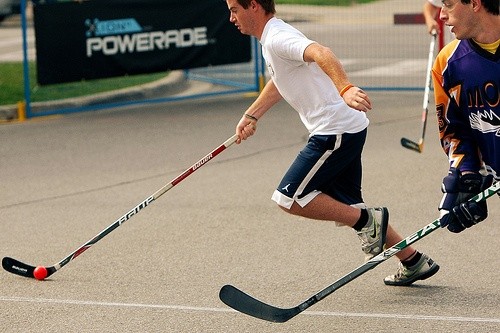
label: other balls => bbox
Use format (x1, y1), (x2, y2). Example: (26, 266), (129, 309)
(34, 266), (47, 279)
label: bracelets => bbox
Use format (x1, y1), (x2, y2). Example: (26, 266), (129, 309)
(340, 84), (353, 97)
(245, 114), (258, 124)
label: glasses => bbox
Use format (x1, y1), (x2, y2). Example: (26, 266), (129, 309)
(440, 0), (461, 12)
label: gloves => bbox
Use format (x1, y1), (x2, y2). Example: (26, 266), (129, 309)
(438, 169), (493, 233)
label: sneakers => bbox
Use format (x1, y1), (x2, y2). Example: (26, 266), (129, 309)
(352, 207), (389, 262)
(384, 253), (439, 286)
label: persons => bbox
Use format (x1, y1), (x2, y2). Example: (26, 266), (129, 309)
(424, 0), (442, 32)
(432, 0), (500, 234)
(226, 0), (440, 286)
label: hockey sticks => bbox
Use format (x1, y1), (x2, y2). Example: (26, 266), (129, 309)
(400, 27), (436, 154)
(1, 123), (256, 279)
(220, 181), (500, 324)
